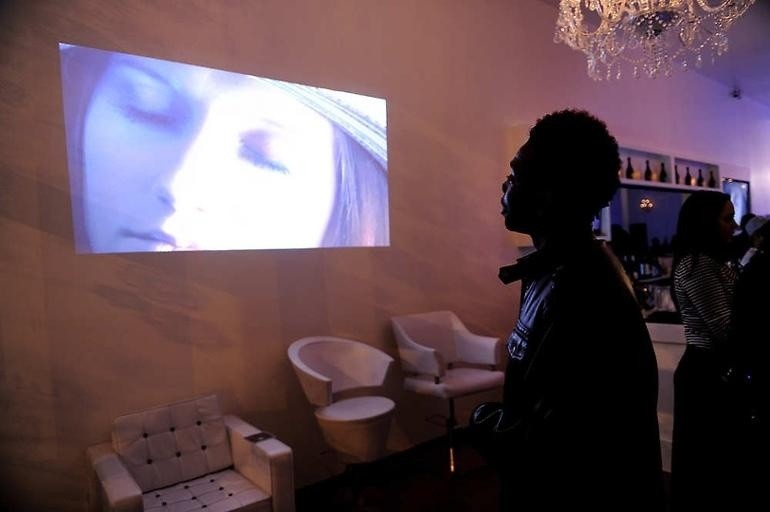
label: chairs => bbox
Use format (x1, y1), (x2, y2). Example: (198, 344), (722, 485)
(391, 309), (505, 473)
(287, 335), (396, 463)
(86, 393), (296, 512)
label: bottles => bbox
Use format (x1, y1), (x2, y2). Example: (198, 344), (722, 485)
(675, 165), (680, 184)
(708, 171), (716, 187)
(644, 160), (651, 180)
(697, 169), (704, 186)
(660, 162), (666, 182)
(626, 157), (633, 179)
(685, 167), (691, 185)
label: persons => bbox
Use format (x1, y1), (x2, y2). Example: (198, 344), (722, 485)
(671, 191), (745, 503)
(498, 112), (663, 503)
(66, 45), (390, 254)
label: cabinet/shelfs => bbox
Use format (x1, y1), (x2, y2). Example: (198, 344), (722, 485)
(592, 145), (723, 319)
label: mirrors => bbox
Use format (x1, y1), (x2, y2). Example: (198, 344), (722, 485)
(722, 178), (750, 226)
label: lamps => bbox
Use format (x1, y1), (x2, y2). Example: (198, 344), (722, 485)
(639, 198), (654, 215)
(550, 0), (754, 83)
